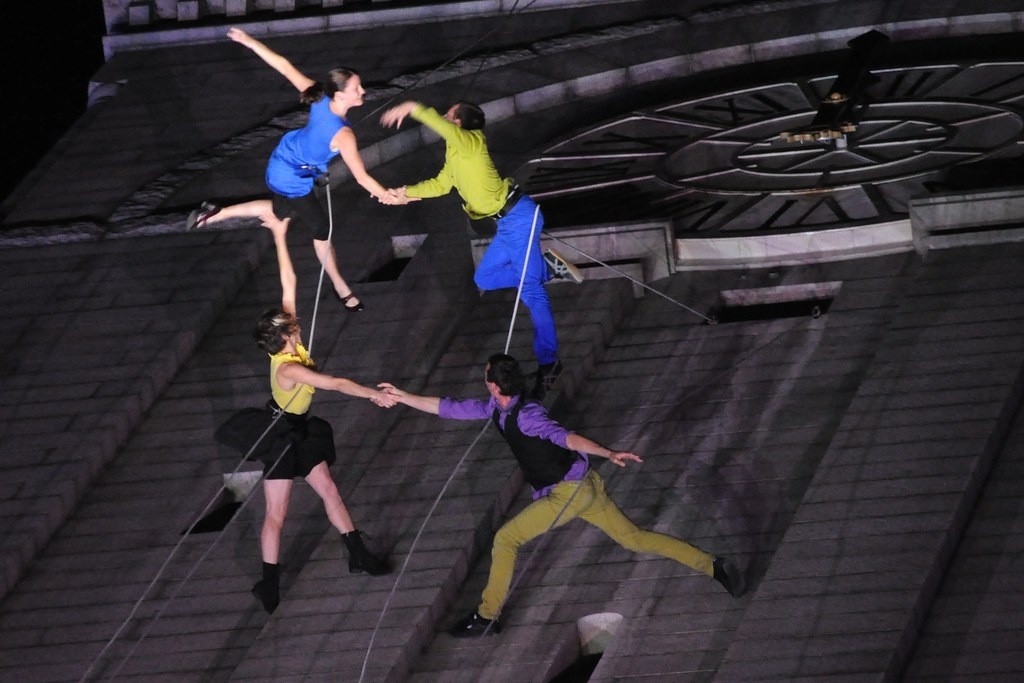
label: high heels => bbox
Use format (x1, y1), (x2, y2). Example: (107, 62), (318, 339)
(349, 556), (390, 576)
(187, 201), (221, 233)
(252, 581), (278, 615)
(334, 290), (364, 312)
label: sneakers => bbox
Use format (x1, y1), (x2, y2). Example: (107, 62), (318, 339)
(542, 249), (583, 285)
(533, 359), (564, 399)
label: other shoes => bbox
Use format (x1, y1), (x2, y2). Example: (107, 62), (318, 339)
(713, 558), (746, 597)
(449, 612), (500, 639)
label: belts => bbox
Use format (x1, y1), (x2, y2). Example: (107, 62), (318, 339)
(492, 187), (522, 221)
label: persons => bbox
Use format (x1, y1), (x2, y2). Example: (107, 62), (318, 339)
(370, 354), (748, 639)
(370, 101), (582, 393)
(212, 213), (394, 615)
(186, 27), (422, 313)
